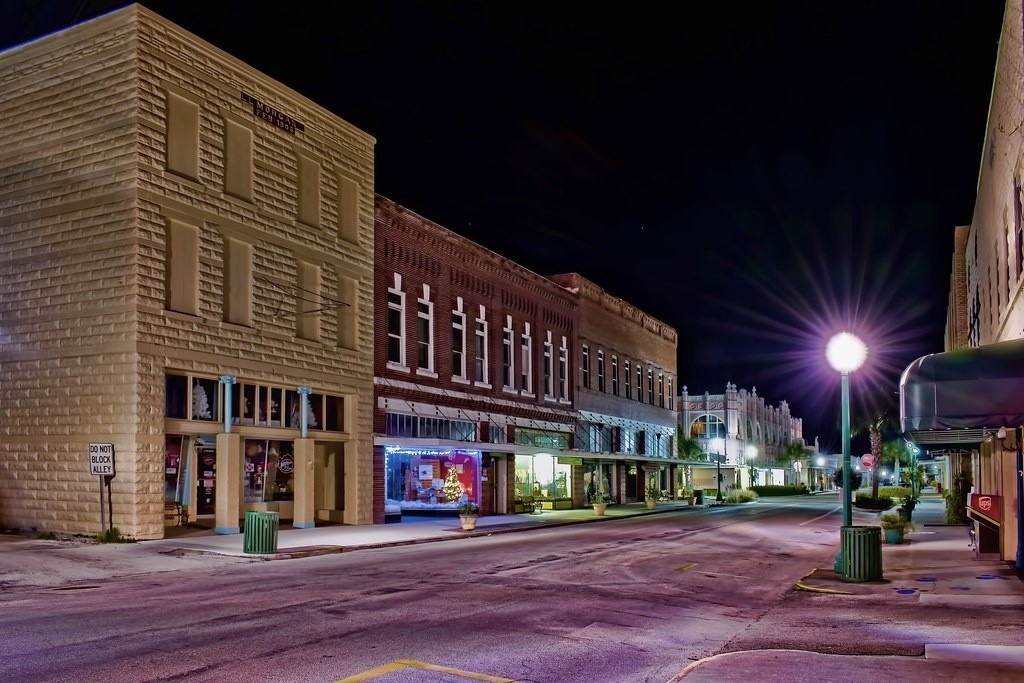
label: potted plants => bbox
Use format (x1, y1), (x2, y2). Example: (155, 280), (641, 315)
(458, 501), (478, 530)
(588, 491), (609, 516)
(687, 492), (698, 506)
(881, 495), (920, 545)
(644, 485), (661, 510)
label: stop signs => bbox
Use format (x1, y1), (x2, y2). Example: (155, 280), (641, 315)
(861, 454), (873, 467)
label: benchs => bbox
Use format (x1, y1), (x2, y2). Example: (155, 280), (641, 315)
(520, 495), (543, 514)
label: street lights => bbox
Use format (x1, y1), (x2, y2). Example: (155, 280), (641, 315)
(818, 457), (826, 491)
(824, 331), (868, 576)
(711, 437), (726, 501)
(745, 444), (757, 488)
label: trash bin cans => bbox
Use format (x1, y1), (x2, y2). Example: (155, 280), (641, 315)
(840, 526), (882, 582)
(694, 490), (704, 505)
(243, 511), (279, 554)
